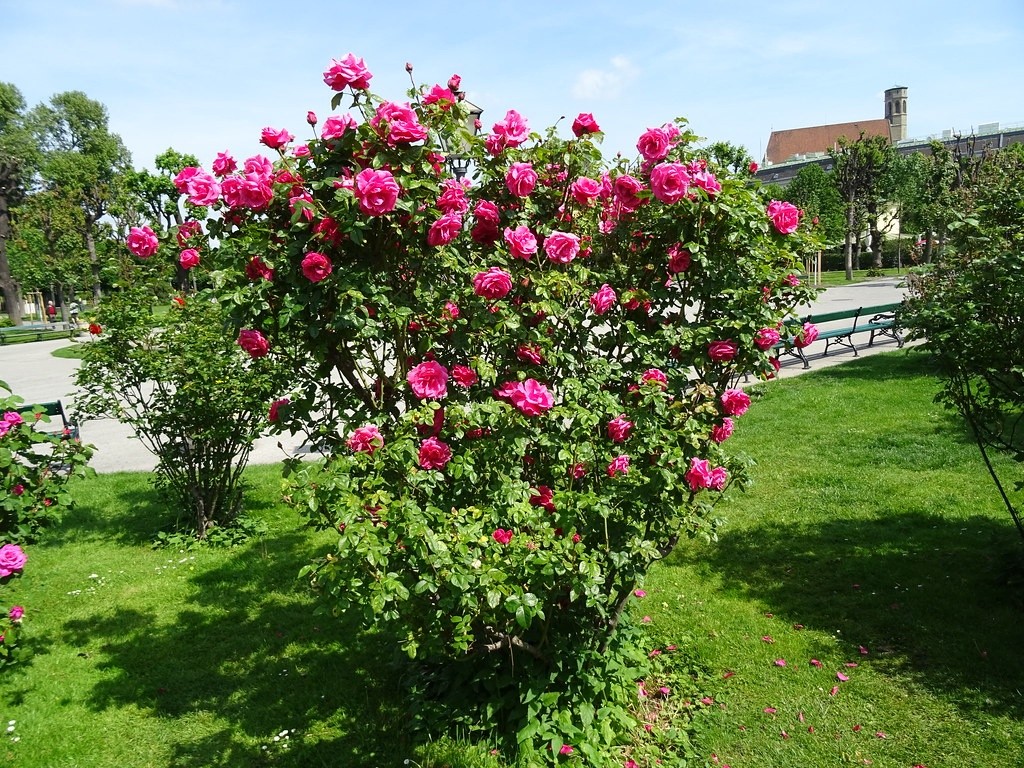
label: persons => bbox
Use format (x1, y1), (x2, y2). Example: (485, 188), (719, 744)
(70, 300), (79, 324)
(46, 301), (57, 319)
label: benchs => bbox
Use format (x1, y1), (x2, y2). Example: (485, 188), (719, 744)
(743, 301), (907, 384)
(17, 401), (80, 441)
(0, 321), (75, 346)
